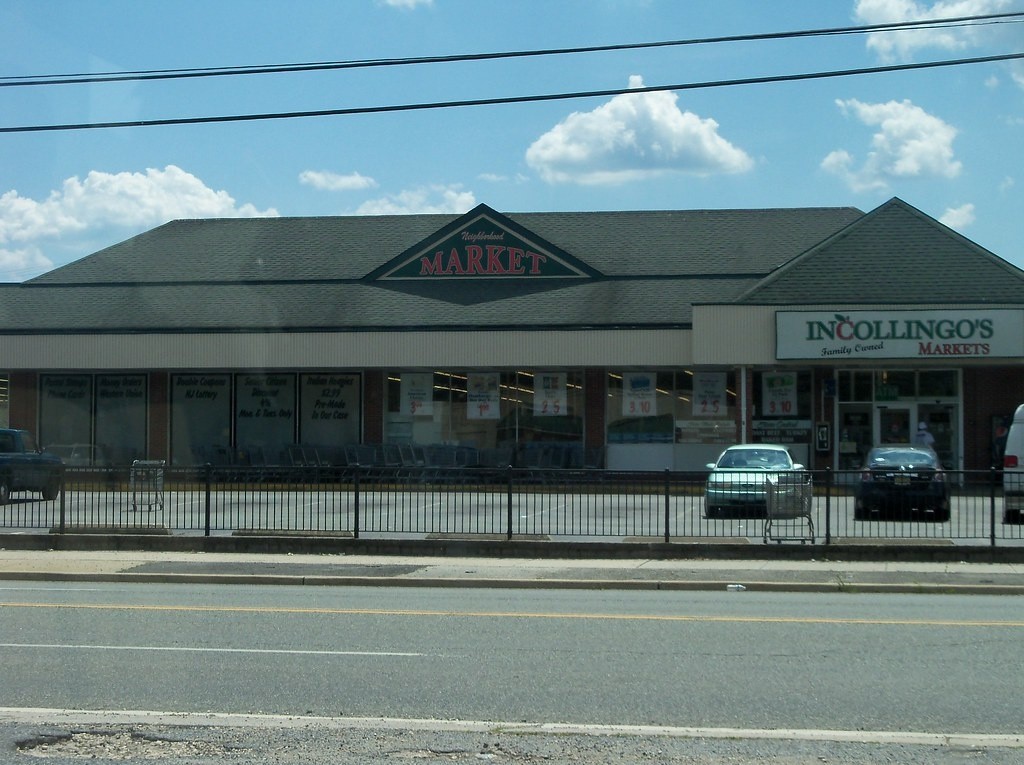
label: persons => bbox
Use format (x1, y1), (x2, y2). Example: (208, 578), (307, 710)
(913, 421), (935, 445)
(884, 422), (908, 444)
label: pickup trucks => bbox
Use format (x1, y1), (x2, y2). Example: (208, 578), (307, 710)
(0, 428), (67, 503)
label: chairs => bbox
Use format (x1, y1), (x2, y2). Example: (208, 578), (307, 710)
(768, 455), (784, 466)
(224, 444), (609, 485)
(734, 459), (747, 468)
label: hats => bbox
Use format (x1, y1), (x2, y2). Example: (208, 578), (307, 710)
(919, 422), (927, 429)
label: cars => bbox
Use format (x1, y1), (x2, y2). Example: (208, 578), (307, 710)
(701, 443), (814, 520)
(34, 443), (105, 492)
(853, 444), (952, 523)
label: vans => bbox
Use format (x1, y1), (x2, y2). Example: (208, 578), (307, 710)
(1000, 404), (1024, 524)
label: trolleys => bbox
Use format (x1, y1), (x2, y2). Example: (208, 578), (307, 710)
(763, 467), (816, 544)
